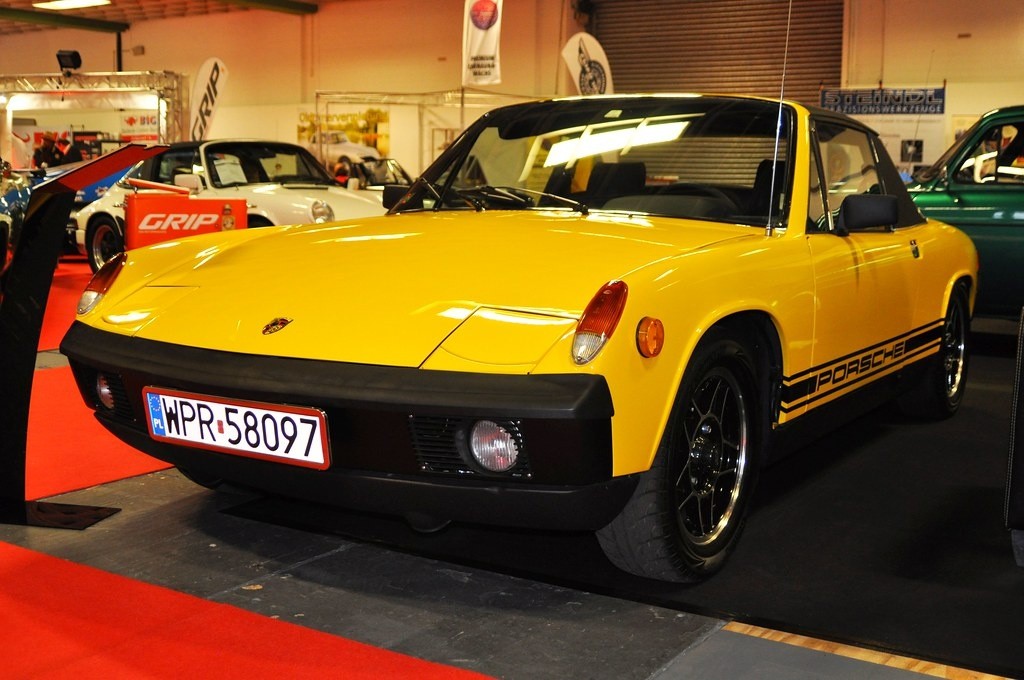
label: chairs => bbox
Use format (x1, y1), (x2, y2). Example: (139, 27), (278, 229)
(748, 159), (785, 215)
(568, 162), (646, 210)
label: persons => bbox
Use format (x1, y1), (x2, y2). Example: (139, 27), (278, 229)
(51, 131), (86, 167)
(331, 162), (354, 186)
(29, 130), (58, 172)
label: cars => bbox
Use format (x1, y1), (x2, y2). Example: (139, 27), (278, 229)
(69, 136), (447, 276)
(1, 135), (185, 267)
(331, 157), (448, 214)
(900, 103), (1023, 320)
(60, 85), (977, 589)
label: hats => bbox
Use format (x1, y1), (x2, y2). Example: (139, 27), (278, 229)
(41, 134), (55, 142)
